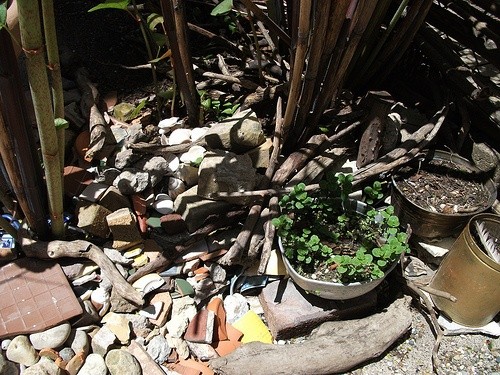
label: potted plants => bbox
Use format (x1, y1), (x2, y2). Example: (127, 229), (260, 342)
(272, 171), (411, 299)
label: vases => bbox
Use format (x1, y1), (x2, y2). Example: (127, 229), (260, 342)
(391, 149), (498, 238)
